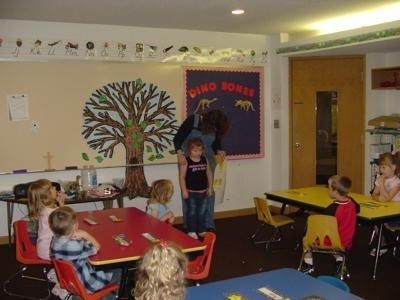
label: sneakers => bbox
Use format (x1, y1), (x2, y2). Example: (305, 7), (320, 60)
(43, 266), (59, 284)
(188, 232), (198, 239)
(370, 248), (388, 257)
(199, 232), (206, 237)
(51, 283), (72, 300)
(299, 262), (314, 274)
(336, 260), (347, 277)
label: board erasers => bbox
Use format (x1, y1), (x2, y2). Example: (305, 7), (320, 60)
(13, 169), (27, 174)
(65, 167), (77, 170)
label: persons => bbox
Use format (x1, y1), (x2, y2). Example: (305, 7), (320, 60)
(27, 183), (63, 245)
(49, 206), (123, 300)
(146, 179), (175, 225)
(298, 174), (360, 278)
(369, 153), (400, 256)
(179, 137), (213, 240)
(173, 109), (229, 232)
(28, 179), (69, 299)
(132, 240), (189, 300)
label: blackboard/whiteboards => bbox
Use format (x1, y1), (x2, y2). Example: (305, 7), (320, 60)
(1, 60), (264, 175)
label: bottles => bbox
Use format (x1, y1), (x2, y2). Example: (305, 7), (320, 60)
(68, 186), (82, 198)
(82, 165), (98, 190)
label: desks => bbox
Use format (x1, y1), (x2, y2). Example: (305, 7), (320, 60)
(0, 185), (128, 254)
(73, 207), (207, 298)
(265, 185), (400, 280)
(186, 267), (366, 300)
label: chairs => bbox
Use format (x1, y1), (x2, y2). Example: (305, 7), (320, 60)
(251, 196), (300, 248)
(318, 275), (350, 292)
(297, 215), (346, 280)
(368, 224), (400, 256)
(53, 258), (120, 300)
(3, 220), (52, 299)
(176, 231), (216, 286)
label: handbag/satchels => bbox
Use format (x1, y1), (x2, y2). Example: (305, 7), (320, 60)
(13, 181), (61, 199)
